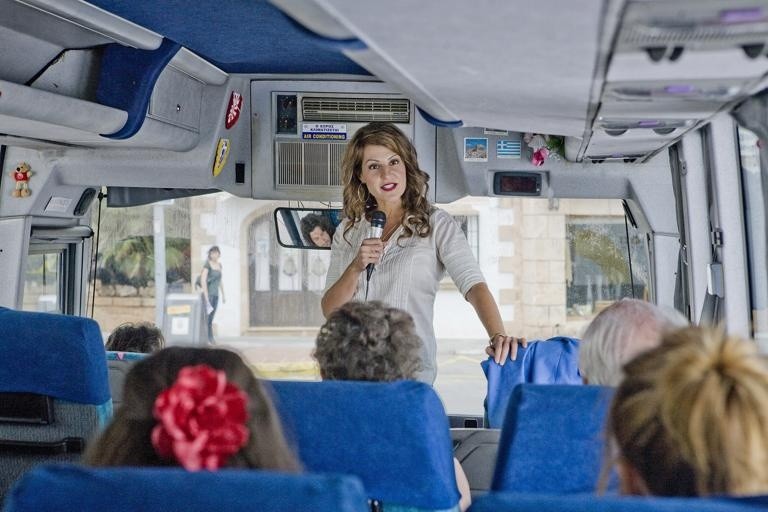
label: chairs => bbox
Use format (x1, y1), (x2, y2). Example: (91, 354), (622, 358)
(253, 377), (461, 510)
(482, 334), (584, 428)
(1, 456), (369, 512)
(462, 490), (767, 512)
(105, 348), (150, 404)
(489, 380), (620, 491)
(1, 306), (115, 509)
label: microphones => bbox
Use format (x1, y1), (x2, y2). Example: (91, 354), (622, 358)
(365, 211), (386, 280)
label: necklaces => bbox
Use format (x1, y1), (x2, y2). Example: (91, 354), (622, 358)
(383, 213), (405, 241)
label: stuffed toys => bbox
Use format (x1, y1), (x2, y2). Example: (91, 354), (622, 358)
(8, 162), (35, 197)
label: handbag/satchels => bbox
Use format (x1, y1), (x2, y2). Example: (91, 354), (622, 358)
(195, 276), (213, 289)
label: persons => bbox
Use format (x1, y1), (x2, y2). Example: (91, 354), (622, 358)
(301, 213), (333, 248)
(200, 246), (226, 344)
(313, 300), (473, 511)
(577, 295), (689, 386)
(105, 319), (166, 355)
(85, 345), (305, 471)
(597, 316), (768, 497)
(321, 120), (527, 389)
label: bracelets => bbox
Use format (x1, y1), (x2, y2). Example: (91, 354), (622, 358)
(490, 333), (504, 348)
